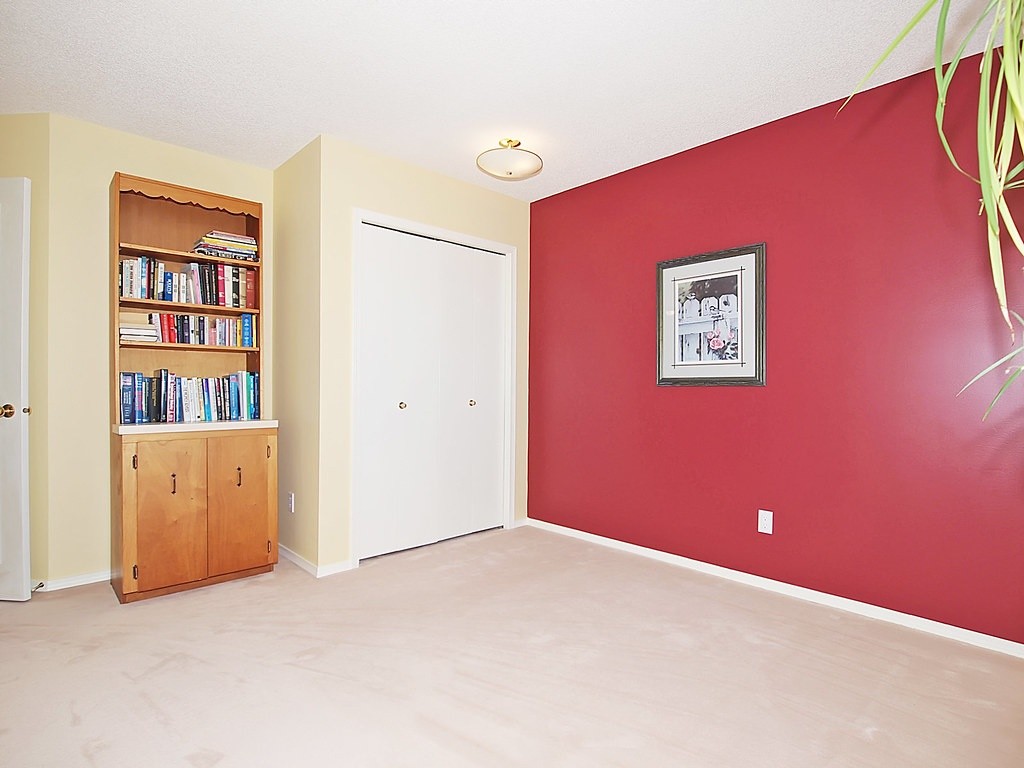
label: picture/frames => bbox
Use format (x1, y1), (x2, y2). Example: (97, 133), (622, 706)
(655, 242), (768, 387)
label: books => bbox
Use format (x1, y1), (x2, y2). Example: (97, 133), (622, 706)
(119, 314), (257, 350)
(190, 230), (258, 263)
(119, 255), (257, 309)
(119, 368), (260, 424)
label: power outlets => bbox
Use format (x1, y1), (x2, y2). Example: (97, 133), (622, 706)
(758, 509), (773, 534)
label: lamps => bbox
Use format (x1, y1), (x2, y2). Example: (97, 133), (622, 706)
(475, 137), (543, 182)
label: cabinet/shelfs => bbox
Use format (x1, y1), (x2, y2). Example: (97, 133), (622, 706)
(110, 171), (279, 435)
(110, 427), (280, 604)
